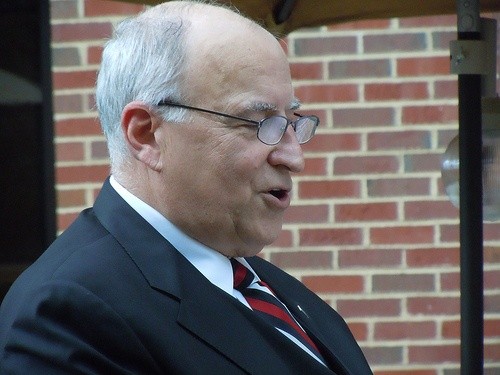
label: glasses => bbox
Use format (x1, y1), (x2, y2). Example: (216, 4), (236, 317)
(156, 99), (320, 146)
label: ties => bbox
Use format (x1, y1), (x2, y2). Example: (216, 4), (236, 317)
(228, 257), (330, 371)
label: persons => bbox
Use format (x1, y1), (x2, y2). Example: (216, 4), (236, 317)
(0, 0), (375, 375)
